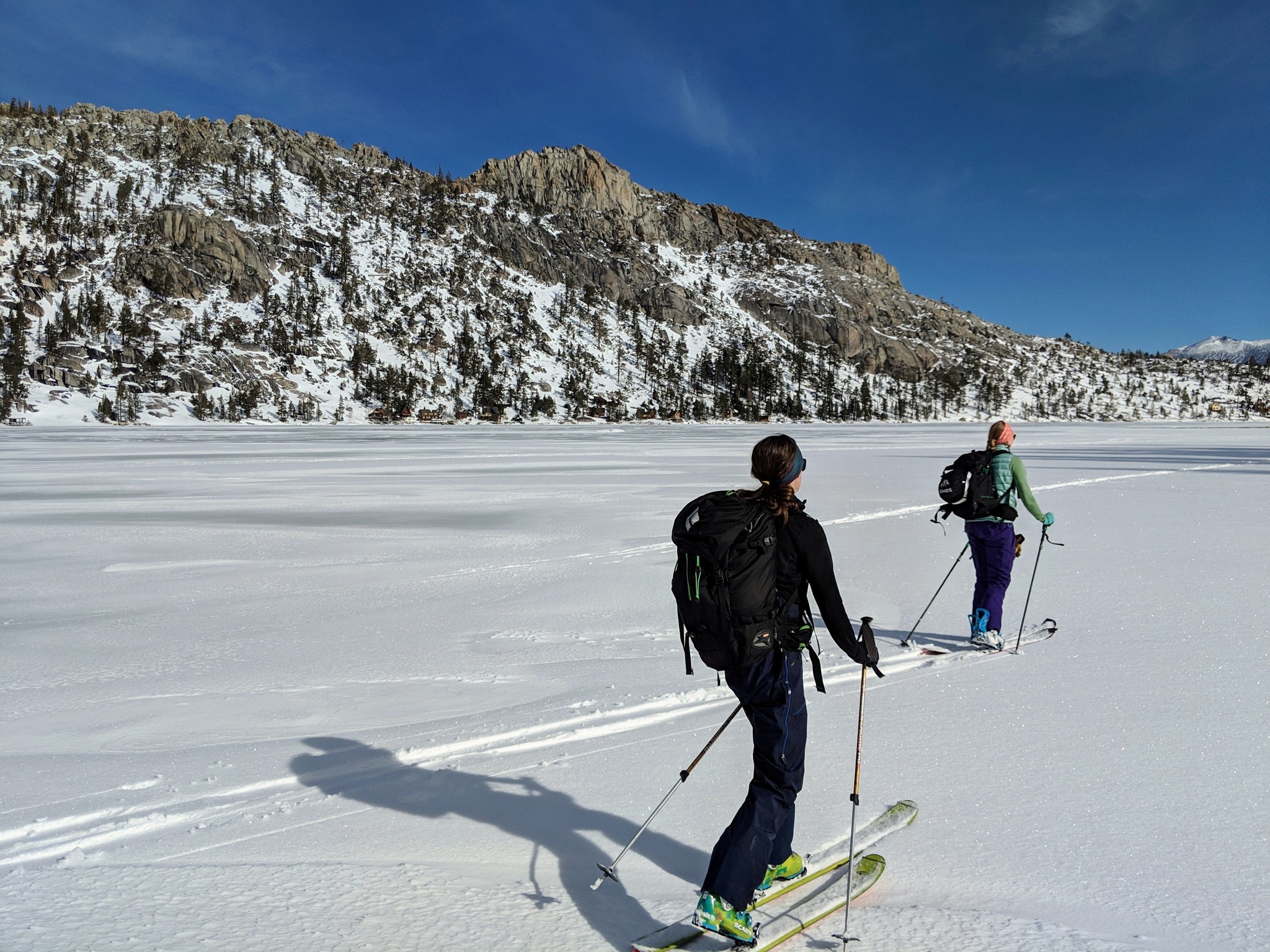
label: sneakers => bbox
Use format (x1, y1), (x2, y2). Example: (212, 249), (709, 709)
(756, 851), (805, 891)
(971, 630), (1004, 649)
(692, 889), (755, 943)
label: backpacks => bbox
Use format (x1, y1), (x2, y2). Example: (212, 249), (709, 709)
(671, 490), (826, 694)
(930, 449), (1018, 536)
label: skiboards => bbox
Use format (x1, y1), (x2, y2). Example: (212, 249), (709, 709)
(626, 798), (918, 950)
(919, 617), (1059, 665)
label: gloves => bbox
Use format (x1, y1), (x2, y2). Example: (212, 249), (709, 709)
(1043, 512), (1054, 525)
(855, 641), (879, 667)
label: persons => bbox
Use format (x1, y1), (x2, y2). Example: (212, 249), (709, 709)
(689, 434), (880, 947)
(964, 420), (1055, 650)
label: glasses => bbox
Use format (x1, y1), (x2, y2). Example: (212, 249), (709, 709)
(802, 459), (807, 471)
(1013, 434), (1016, 440)
